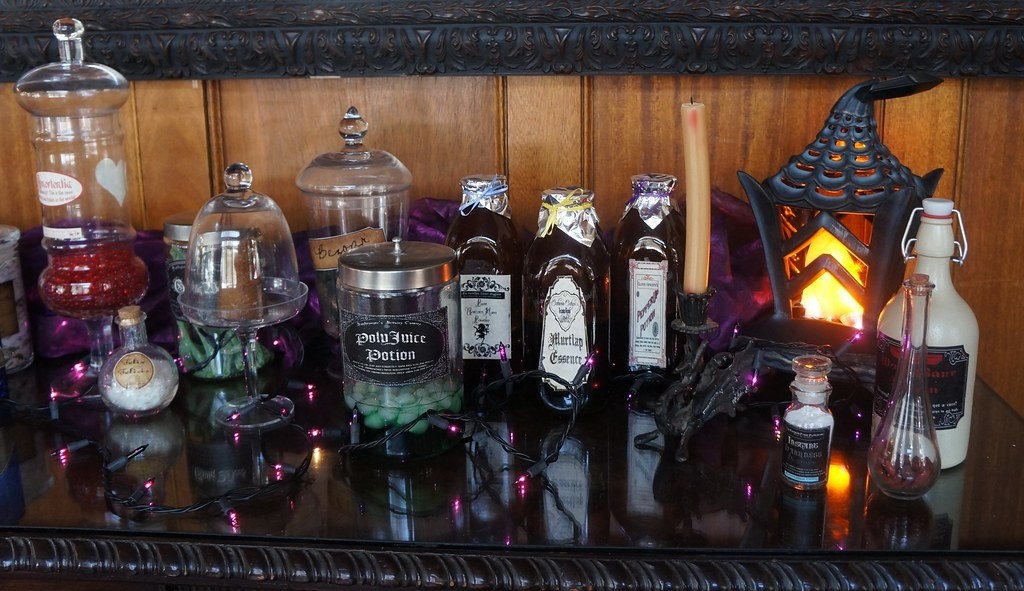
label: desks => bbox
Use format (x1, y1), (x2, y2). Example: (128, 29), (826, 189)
(1, 252), (1024, 588)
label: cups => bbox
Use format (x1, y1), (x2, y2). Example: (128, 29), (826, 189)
(0, 224), (34, 372)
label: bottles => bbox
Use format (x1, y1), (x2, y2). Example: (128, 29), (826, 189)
(97, 306), (181, 417)
(520, 186), (612, 394)
(611, 173), (680, 374)
(867, 273), (941, 499)
(444, 174), (521, 410)
(872, 197), (979, 468)
(783, 354), (834, 490)
